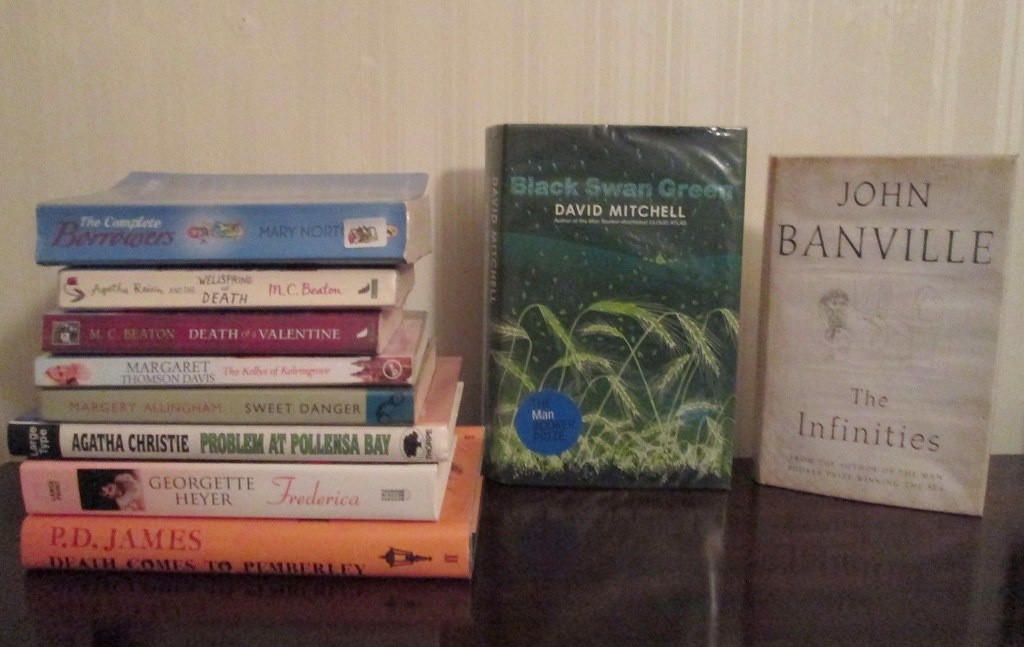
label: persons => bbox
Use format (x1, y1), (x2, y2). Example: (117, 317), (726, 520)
(91, 472), (144, 511)
(42, 359), (104, 385)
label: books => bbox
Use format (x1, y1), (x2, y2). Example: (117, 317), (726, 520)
(754, 152), (1017, 516)
(477, 122), (749, 490)
(9, 169), (490, 580)
